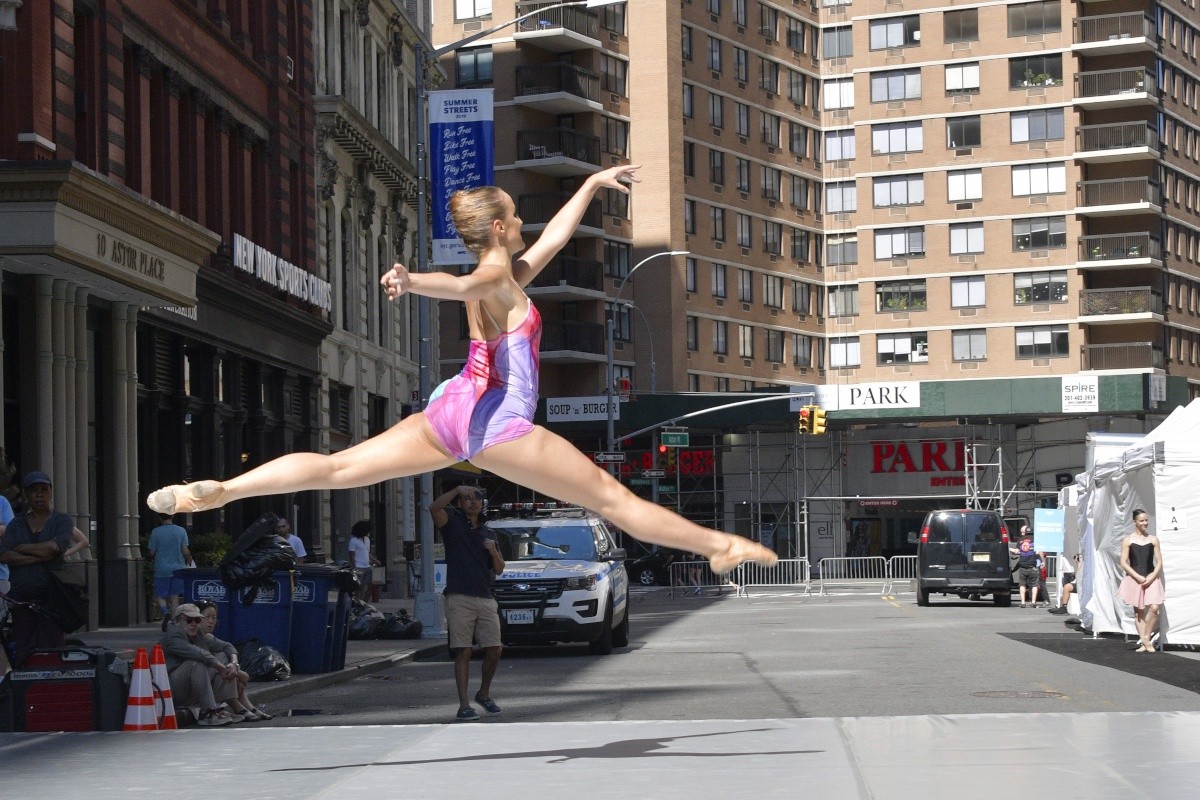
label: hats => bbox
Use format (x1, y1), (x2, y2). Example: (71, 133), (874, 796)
(1026, 527), (1033, 534)
(23, 471), (53, 488)
(174, 604), (205, 618)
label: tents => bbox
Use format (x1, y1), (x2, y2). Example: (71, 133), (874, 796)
(1058, 397), (1200, 652)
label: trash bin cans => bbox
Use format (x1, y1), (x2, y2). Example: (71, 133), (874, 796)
(172, 568), (231, 643)
(219, 512), (292, 665)
(289, 565), (360, 674)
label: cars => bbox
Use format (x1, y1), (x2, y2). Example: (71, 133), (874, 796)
(625, 546), (709, 586)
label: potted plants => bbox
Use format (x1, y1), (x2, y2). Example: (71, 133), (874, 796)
(1092, 247), (1107, 260)
(1135, 71), (1143, 86)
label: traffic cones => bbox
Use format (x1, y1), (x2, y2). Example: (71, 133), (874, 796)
(148, 645), (179, 730)
(122, 648), (159, 731)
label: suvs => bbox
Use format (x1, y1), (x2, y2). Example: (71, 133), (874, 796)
(483, 503), (630, 654)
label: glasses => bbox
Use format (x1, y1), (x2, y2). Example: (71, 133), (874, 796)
(182, 617), (202, 624)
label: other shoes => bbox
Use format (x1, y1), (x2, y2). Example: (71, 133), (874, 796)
(456, 707), (480, 720)
(1030, 604), (1036, 608)
(239, 708), (272, 721)
(160, 612), (171, 632)
(197, 707), (246, 725)
(475, 690), (501, 715)
(1021, 604), (1025, 608)
(1049, 607), (1067, 614)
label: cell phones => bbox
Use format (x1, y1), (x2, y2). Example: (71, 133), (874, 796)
(473, 489), (487, 498)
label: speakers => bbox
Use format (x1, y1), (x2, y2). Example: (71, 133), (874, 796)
(0, 646), (130, 731)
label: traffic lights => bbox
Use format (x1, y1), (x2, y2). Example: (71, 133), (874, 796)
(658, 444), (668, 467)
(668, 446), (677, 467)
(798, 408), (810, 436)
(812, 408), (828, 436)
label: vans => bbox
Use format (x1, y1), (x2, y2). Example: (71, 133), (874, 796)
(907, 510), (1030, 607)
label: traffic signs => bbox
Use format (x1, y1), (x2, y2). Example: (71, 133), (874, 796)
(593, 452), (626, 465)
(641, 468), (667, 479)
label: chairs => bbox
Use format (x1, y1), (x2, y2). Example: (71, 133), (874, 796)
(528, 144), (545, 159)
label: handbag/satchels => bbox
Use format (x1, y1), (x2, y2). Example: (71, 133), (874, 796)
(48, 575), (89, 635)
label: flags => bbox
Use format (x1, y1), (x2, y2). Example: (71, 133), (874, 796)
(427, 89), (494, 265)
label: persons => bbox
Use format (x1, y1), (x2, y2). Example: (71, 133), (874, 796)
(147, 162), (779, 574)
(349, 520), (379, 601)
(274, 517), (308, 564)
(150, 604), (245, 725)
(430, 485), (506, 718)
(0, 472), (74, 666)
(0, 496), (15, 675)
(29, 500), (89, 556)
(1009, 526), (1051, 608)
(146, 515), (192, 620)
(1118, 509), (1164, 652)
(198, 600), (271, 722)
(1048, 554), (1079, 614)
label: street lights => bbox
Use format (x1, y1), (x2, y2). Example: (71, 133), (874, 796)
(607, 251), (692, 477)
